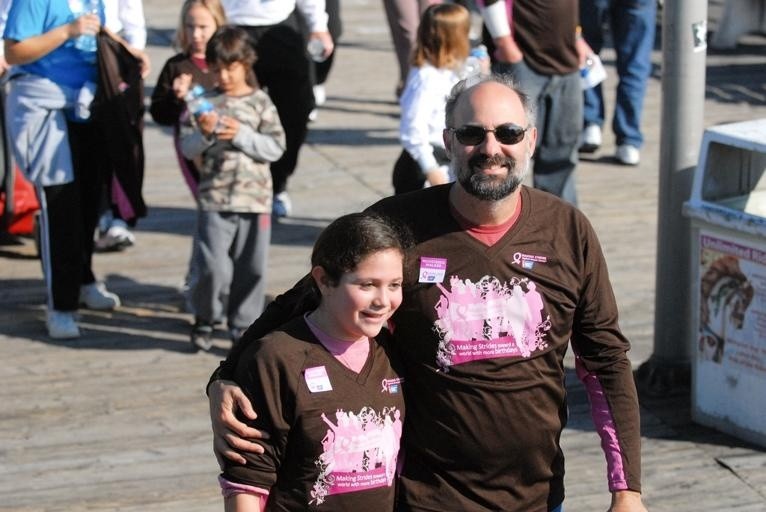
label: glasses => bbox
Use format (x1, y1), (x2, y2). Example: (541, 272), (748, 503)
(448, 122), (530, 145)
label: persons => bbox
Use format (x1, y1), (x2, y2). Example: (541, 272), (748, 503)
(295, 0), (344, 120)
(211, 212), (407, 511)
(474, 1), (595, 209)
(379, 0), (445, 105)
(578, 0), (658, 166)
(149, 1), (230, 317)
(389, 2), (491, 197)
(1, 0), (149, 340)
(220, 0), (334, 218)
(178, 22), (287, 350)
(204, 74), (649, 512)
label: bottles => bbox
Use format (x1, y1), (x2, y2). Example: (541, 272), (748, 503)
(580, 58), (593, 77)
(183, 84), (225, 135)
(461, 49), (486, 80)
(74, 0), (98, 52)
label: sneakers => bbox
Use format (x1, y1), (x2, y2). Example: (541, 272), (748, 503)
(192, 323), (214, 352)
(94, 226), (135, 251)
(78, 281), (119, 310)
(615, 145), (640, 165)
(577, 125), (602, 153)
(45, 313), (81, 339)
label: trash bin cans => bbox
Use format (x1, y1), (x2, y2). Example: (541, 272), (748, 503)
(681, 118), (766, 448)
